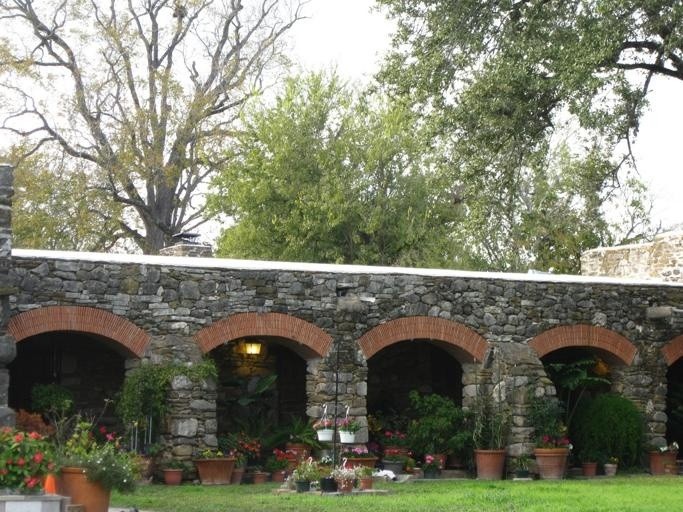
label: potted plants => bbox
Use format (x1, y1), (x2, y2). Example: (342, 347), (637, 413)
(471, 393), (509, 480)
(408, 390), (471, 470)
(647, 447), (677, 475)
(581, 449), (618, 478)
(161, 460), (185, 484)
(192, 449), (237, 485)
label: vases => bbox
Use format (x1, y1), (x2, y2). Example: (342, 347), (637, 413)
(60, 466), (111, 512)
(534, 448), (569, 479)
(0, 493), (63, 512)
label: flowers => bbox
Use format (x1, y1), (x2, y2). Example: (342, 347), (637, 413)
(0, 427), (59, 495)
(55, 418), (136, 495)
(534, 419), (572, 448)
(236, 417), (446, 493)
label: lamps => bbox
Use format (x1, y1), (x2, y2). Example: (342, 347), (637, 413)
(243, 337), (263, 354)
